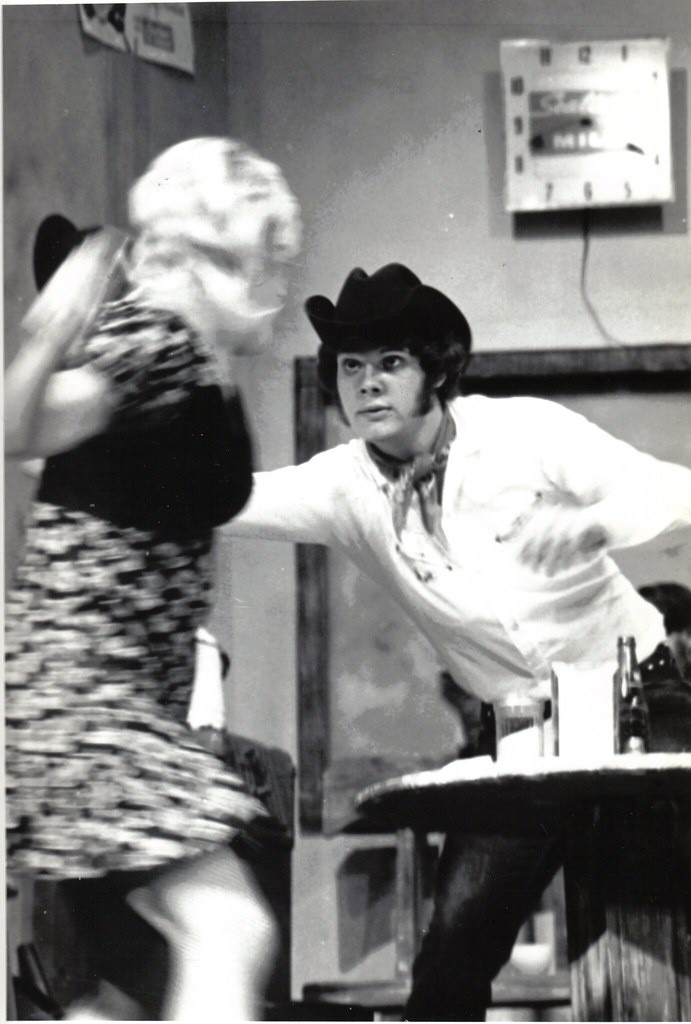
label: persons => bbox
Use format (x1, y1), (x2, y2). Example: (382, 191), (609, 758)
(5, 139), (301, 1021)
(21, 265), (691, 1021)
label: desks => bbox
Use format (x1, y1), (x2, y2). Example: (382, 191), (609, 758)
(352, 752), (691, 1022)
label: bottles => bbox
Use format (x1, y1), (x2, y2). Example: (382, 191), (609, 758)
(612, 636), (649, 755)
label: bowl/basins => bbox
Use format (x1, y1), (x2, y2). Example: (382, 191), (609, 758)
(511, 945), (550, 976)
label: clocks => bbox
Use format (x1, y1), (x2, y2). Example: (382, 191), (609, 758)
(499, 36), (675, 212)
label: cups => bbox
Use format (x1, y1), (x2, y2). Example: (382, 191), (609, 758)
(494, 695), (543, 761)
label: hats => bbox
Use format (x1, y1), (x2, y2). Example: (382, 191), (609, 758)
(305, 262), (472, 352)
